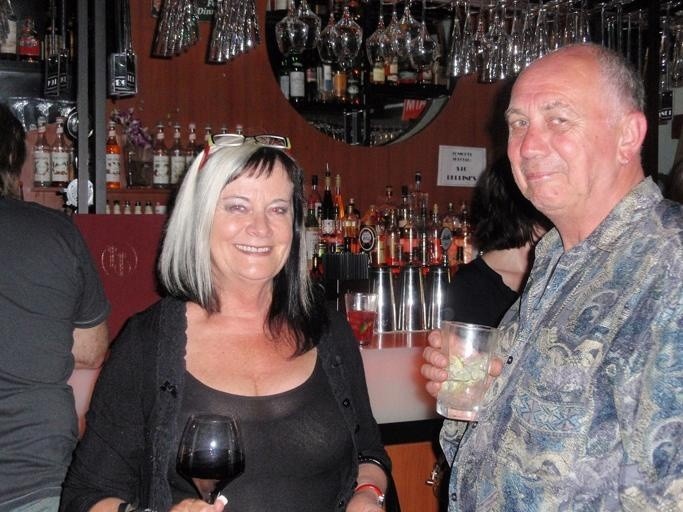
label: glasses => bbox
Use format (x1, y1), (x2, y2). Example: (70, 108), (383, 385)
(209, 131), (292, 152)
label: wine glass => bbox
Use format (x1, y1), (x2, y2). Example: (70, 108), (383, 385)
(317, 6), (335, 62)
(668, 25), (683, 89)
(330, 5), (362, 64)
(275, 1), (309, 56)
(289, 1), (321, 53)
(446, 2), (514, 82)
(175, 413), (246, 505)
(151, 2), (200, 57)
(391, 6), (421, 59)
(366, 6), (386, 67)
(409, 15), (438, 73)
(514, 0), (591, 78)
(207, 1), (260, 65)
(379, 8), (398, 65)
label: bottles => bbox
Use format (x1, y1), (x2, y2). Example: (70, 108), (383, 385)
(113, 199), (120, 215)
(272, 42), (454, 116)
(123, 200), (131, 213)
(18, 15), (40, 62)
(200, 122), (212, 150)
(187, 123), (200, 168)
(33, 116), (50, 188)
(170, 120), (185, 188)
(51, 116), (69, 187)
(152, 120), (170, 189)
(106, 123), (120, 190)
(106, 199), (111, 213)
(297, 159), (479, 274)
(1, 0), (19, 60)
(217, 122), (229, 135)
(134, 201), (142, 215)
(144, 200), (154, 213)
(234, 124), (244, 138)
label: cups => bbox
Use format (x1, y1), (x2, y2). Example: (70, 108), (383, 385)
(344, 292), (380, 347)
(436, 318), (500, 422)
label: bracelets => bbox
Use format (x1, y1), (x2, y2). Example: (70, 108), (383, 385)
(355, 482), (390, 507)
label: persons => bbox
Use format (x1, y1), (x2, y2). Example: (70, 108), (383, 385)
(420, 42), (683, 512)
(453, 156), (552, 323)
(58, 132), (393, 512)
(0, 104), (109, 512)
